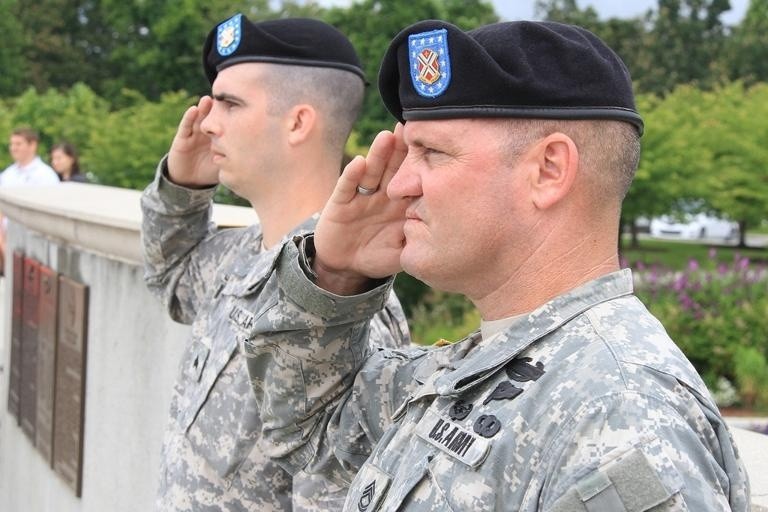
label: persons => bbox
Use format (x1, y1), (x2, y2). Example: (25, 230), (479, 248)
(49, 144), (88, 283)
(1, 129), (62, 277)
(222, 15), (751, 512)
(137, 8), (413, 512)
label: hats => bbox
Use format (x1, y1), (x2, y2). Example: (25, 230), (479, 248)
(201, 15), (371, 89)
(377, 20), (645, 137)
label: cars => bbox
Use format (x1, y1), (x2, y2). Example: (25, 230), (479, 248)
(651, 200), (740, 243)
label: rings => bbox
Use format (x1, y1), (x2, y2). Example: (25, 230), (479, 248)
(355, 183), (381, 196)
